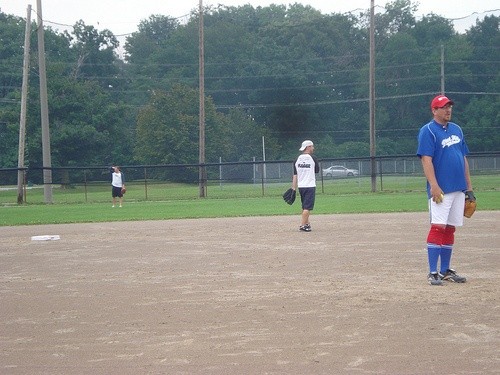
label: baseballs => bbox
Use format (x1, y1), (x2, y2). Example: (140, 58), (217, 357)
(441, 194), (443, 199)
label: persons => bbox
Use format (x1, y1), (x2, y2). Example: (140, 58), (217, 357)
(282, 141), (320, 232)
(110, 166), (125, 208)
(416, 96), (476, 286)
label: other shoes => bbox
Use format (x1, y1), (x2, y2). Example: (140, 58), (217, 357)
(299, 225), (311, 231)
(119, 206), (122, 207)
(112, 205), (115, 207)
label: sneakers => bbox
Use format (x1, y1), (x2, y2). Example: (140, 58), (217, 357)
(438, 269), (466, 283)
(427, 272), (442, 285)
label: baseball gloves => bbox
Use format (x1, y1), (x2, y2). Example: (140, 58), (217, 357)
(464, 194), (477, 218)
(283, 188), (296, 205)
(120, 188), (126, 194)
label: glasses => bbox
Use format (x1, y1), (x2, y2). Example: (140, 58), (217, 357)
(439, 105), (452, 109)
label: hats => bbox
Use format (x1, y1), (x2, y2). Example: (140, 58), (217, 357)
(299, 141), (313, 151)
(431, 95), (454, 108)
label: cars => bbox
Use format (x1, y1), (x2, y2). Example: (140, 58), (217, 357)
(322, 165), (359, 177)
